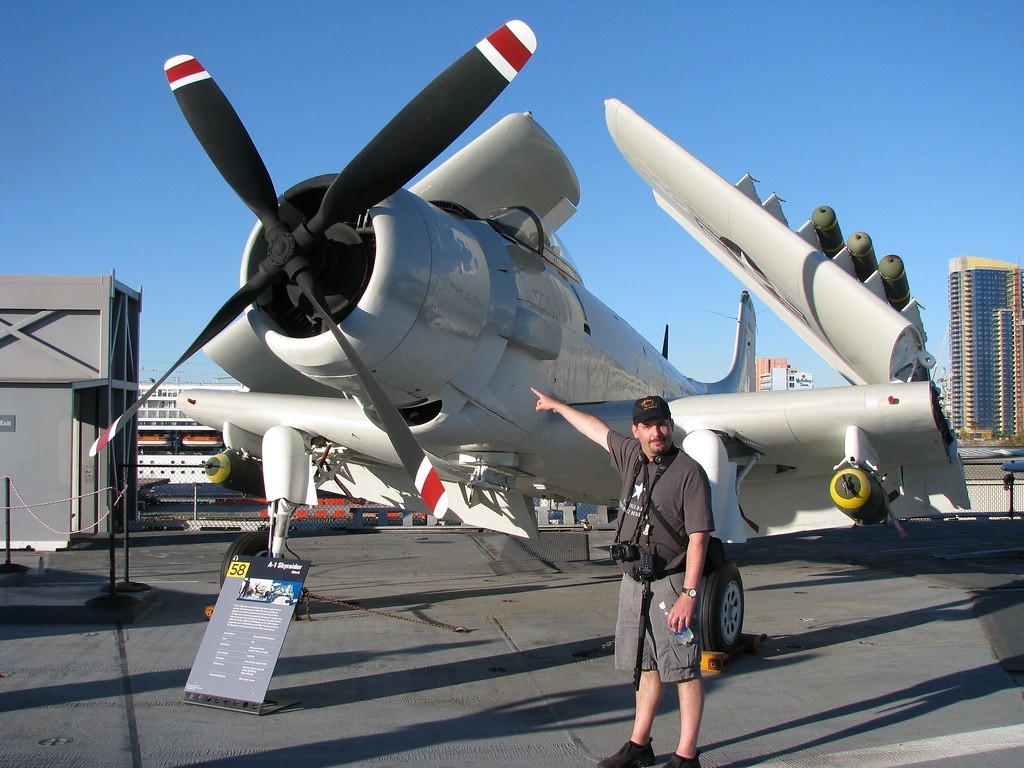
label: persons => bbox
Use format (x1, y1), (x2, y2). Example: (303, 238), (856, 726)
(530, 388), (715, 768)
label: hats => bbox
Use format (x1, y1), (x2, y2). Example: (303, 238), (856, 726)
(632, 396), (671, 422)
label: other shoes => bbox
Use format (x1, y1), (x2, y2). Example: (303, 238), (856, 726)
(661, 751), (700, 768)
(598, 737), (655, 768)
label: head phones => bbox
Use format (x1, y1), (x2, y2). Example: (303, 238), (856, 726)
(638, 441), (673, 465)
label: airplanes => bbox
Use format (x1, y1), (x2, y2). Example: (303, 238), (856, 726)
(71, 20), (976, 667)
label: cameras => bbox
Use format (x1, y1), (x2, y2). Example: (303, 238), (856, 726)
(610, 540), (640, 562)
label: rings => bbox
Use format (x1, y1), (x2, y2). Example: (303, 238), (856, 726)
(678, 619), (684, 622)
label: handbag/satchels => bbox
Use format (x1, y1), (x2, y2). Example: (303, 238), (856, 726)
(701, 537), (726, 577)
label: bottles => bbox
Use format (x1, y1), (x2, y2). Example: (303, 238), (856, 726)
(659, 601), (694, 645)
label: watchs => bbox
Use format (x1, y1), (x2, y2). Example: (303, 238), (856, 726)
(682, 588), (698, 598)
